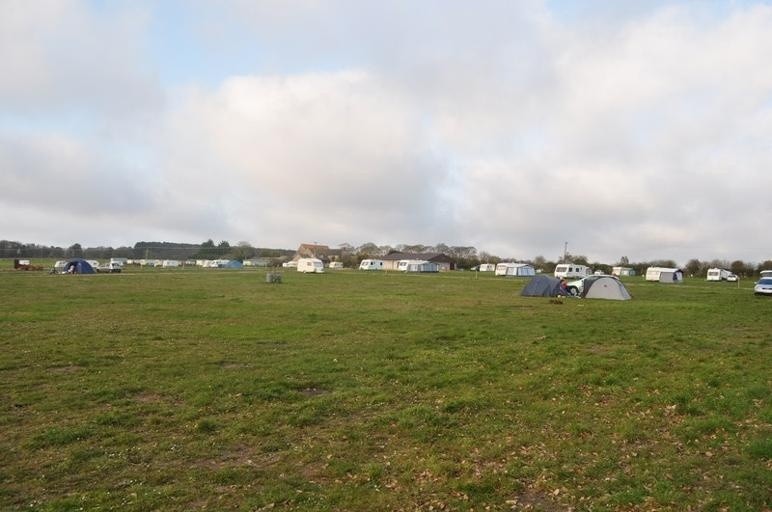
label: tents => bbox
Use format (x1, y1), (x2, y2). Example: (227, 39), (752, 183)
(63, 258), (96, 274)
(579, 276), (632, 300)
(521, 274), (568, 298)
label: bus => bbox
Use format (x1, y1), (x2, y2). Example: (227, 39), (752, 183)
(758, 270), (771, 280)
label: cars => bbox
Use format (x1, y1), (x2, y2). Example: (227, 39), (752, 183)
(753, 277), (771, 296)
(594, 269), (605, 275)
(97, 262), (122, 273)
(54, 254), (255, 270)
(726, 274), (740, 281)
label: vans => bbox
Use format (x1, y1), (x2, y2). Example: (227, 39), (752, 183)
(555, 262), (585, 279)
(297, 256), (326, 275)
(705, 267), (722, 281)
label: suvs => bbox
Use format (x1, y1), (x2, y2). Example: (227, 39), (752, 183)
(562, 273), (619, 297)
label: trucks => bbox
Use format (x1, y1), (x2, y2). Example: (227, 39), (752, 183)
(328, 258), (495, 274)
